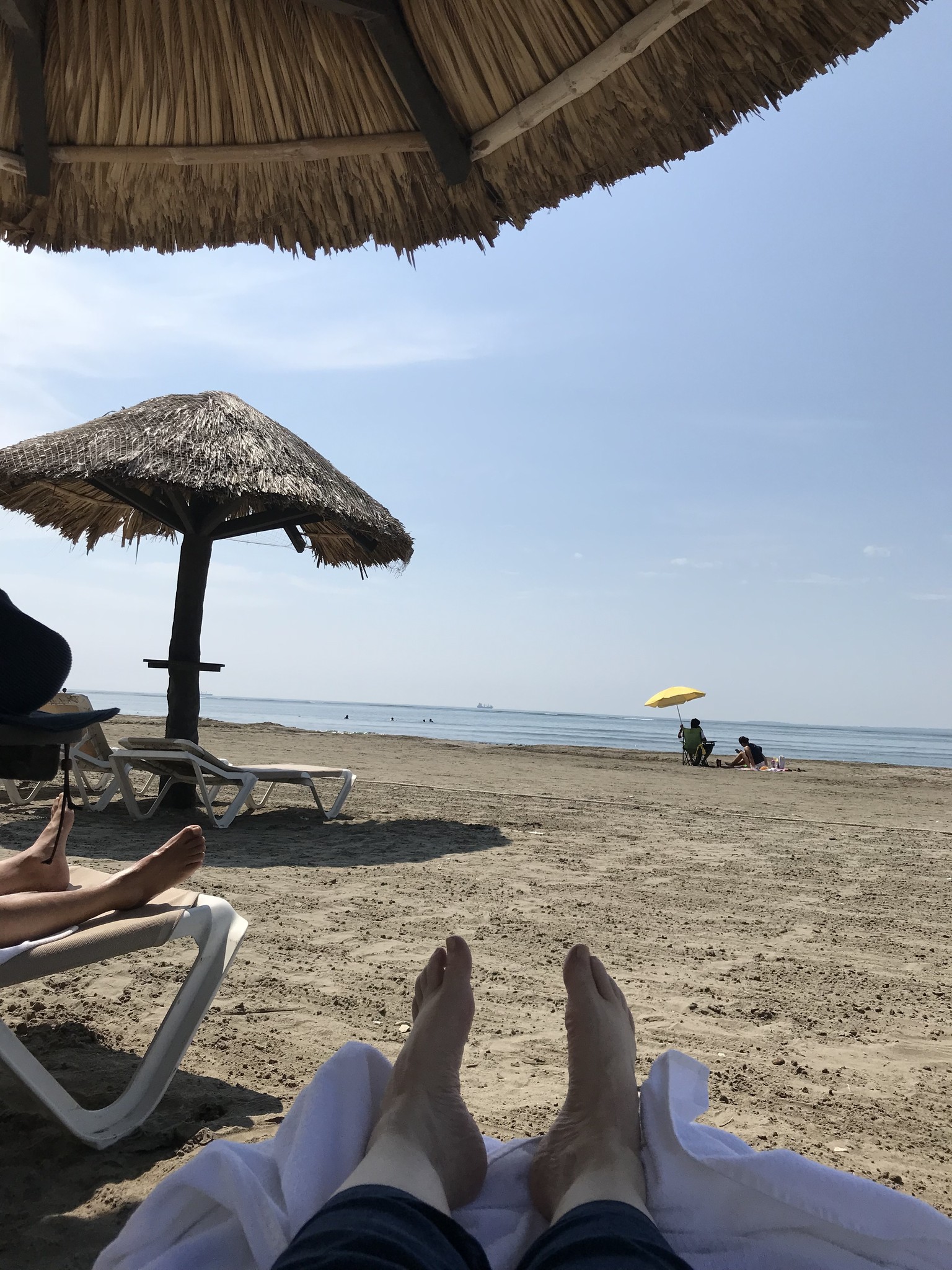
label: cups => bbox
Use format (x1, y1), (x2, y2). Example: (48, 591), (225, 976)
(771, 760), (775, 768)
(716, 759), (722, 767)
(777, 761), (779, 768)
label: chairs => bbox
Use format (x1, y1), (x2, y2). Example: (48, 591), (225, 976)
(0, 729), (131, 814)
(2, 855), (246, 1155)
(679, 726), (718, 766)
(113, 735), (357, 830)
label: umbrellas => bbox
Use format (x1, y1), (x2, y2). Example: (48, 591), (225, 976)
(0, 387), (419, 805)
(644, 685), (706, 733)
(0, 0), (938, 261)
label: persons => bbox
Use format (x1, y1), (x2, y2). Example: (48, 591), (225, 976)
(725, 735), (765, 770)
(344, 715), (349, 719)
(680, 718), (713, 767)
(391, 717), (397, 721)
(678, 718), (703, 765)
(423, 719), (425, 722)
(429, 719), (434, 722)
(268, 924), (696, 1269)
(0, 792), (207, 947)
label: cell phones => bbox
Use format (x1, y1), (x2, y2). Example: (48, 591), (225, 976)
(735, 749), (740, 754)
